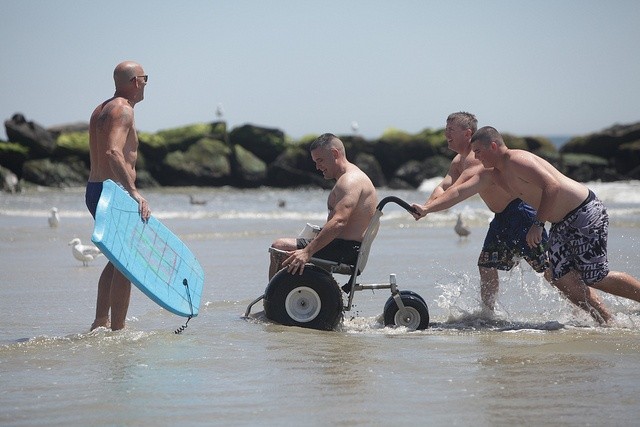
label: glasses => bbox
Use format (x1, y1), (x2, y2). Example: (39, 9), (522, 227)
(130, 75), (148, 81)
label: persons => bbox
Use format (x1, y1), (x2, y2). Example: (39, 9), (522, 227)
(85, 61), (151, 331)
(269, 132), (379, 281)
(409, 126), (640, 332)
(413, 111), (616, 325)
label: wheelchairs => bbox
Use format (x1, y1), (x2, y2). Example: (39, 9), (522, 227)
(240, 197), (429, 332)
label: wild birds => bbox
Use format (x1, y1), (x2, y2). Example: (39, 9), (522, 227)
(455, 213), (470, 240)
(48, 207), (59, 229)
(69, 237), (102, 266)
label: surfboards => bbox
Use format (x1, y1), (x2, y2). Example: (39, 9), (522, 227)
(90, 178), (205, 334)
